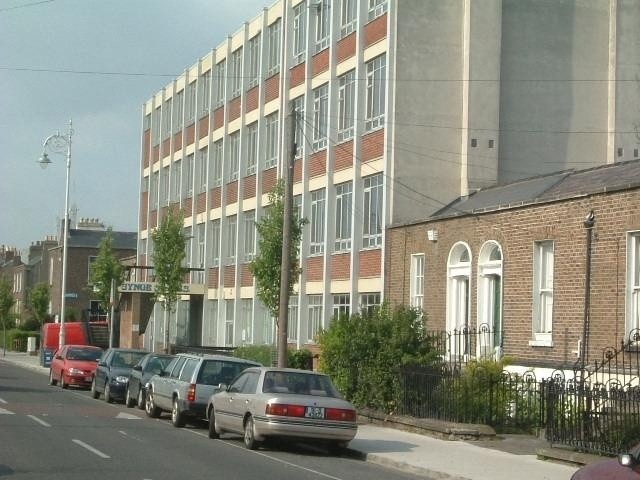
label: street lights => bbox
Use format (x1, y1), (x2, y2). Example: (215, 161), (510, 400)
(35, 114), (76, 354)
